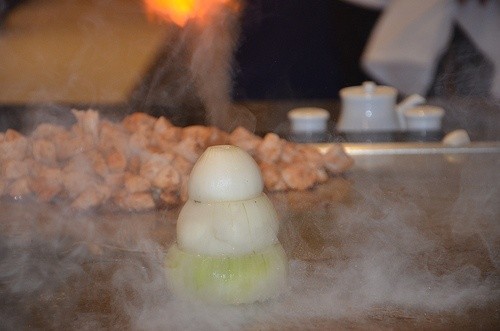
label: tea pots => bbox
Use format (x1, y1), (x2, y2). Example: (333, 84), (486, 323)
(332, 79), (428, 143)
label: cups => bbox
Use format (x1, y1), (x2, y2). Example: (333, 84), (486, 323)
(398, 100), (445, 141)
(287, 105), (330, 144)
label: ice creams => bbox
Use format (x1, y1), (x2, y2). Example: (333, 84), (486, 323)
(160, 143), (288, 303)
